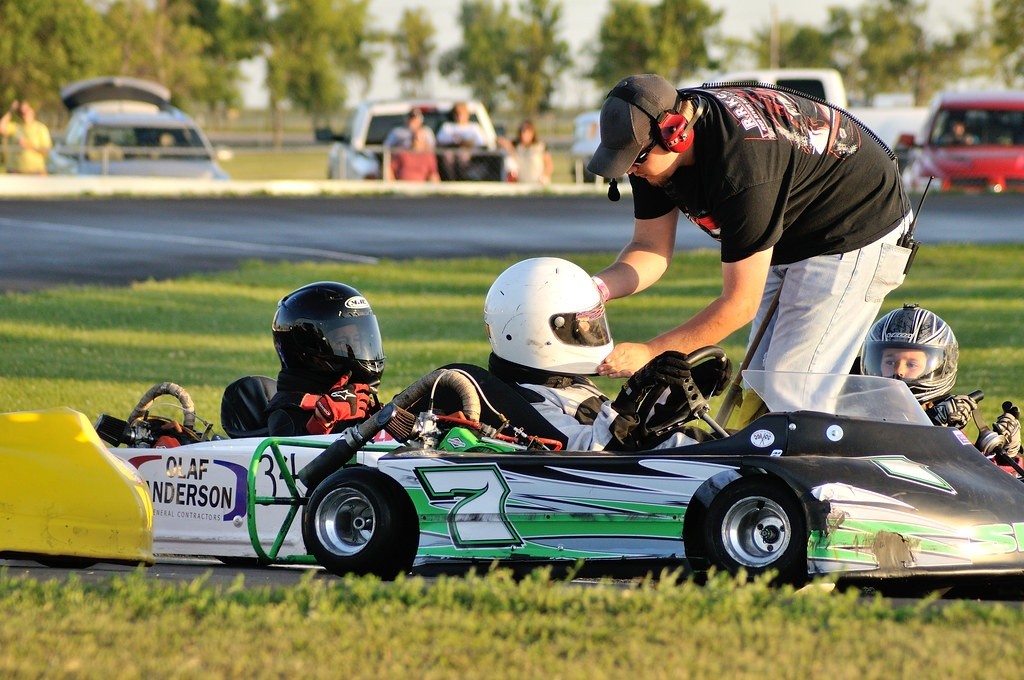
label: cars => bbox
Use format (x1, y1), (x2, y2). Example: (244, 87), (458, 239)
(891, 91), (1024, 198)
(312, 96), (519, 184)
(46, 75), (231, 185)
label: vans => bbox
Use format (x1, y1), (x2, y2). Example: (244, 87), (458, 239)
(574, 67), (921, 186)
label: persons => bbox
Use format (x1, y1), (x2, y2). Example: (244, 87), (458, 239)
(385, 102), (551, 185)
(266, 282), (393, 442)
(938, 119), (973, 145)
(0, 100), (53, 175)
(588, 73), (915, 414)
(859, 306), (1024, 480)
(484, 256), (741, 455)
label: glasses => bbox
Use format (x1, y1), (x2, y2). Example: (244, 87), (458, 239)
(635, 137), (660, 167)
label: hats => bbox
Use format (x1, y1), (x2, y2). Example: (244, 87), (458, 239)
(586, 73), (678, 179)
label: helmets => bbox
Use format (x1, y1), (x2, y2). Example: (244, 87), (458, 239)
(485, 256), (615, 375)
(273, 281), (383, 390)
(860, 307), (958, 402)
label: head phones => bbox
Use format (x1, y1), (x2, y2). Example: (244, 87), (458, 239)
(654, 92), (711, 153)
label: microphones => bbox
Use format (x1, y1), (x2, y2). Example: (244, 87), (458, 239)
(608, 178), (621, 202)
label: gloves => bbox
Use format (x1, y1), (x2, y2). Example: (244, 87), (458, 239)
(689, 353), (733, 397)
(933, 394), (977, 430)
(612, 350), (693, 417)
(992, 412), (1022, 459)
(306, 371), (380, 437)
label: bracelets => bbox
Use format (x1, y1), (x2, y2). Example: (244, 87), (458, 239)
(592, 275), (610, 301)
